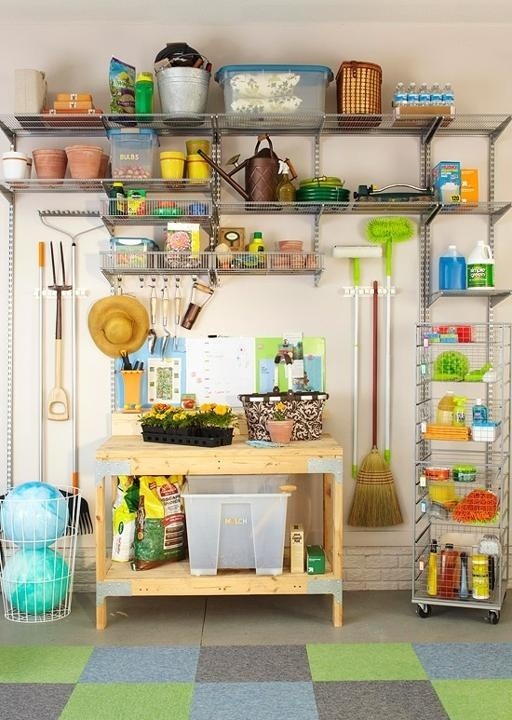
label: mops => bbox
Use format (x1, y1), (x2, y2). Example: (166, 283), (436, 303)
(363, 216), (419, 466)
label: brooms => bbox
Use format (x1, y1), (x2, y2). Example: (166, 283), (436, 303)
(332, 244), (383, 477)
(347, 280), (404, 526)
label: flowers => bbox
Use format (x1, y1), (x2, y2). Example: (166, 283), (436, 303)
(272, 402), (289, 419)
(140, 402), (233, 431)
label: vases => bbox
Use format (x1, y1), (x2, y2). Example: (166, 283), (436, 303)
(5, 139), (213, 191)
(141, 427), (234, 447)
(265, 421), (298, 444)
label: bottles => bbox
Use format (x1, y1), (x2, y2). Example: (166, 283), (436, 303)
(472, 397), (489, 423)
(247, 231), (268, 269)
(438, 542), (459, 599)
(391, 81), (457, 123)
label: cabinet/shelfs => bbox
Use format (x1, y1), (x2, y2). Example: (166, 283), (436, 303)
(410, 113), (512, 623)
(3, 115), (444, 283)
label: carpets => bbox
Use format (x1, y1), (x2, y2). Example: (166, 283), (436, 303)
(0, 643), (509, 720)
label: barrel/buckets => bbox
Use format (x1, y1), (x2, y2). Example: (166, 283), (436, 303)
(157, 52), (212, 127)
(466, 240), (496, 290)
(134, 71), (154, 122)
(439, 245), (467, 291)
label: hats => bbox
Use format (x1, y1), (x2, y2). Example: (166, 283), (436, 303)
(87, 294), (150, 359)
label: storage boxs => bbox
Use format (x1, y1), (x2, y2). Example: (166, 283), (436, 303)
(214, 64), (335, 131)
(107, 126), (159, 183)
(107, 235), (156, 269)
(177, 479), (290, 578)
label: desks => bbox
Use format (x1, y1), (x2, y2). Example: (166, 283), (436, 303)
(91, 436), (345, 629)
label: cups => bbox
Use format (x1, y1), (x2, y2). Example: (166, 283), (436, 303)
(457, 325), (471, 343)
